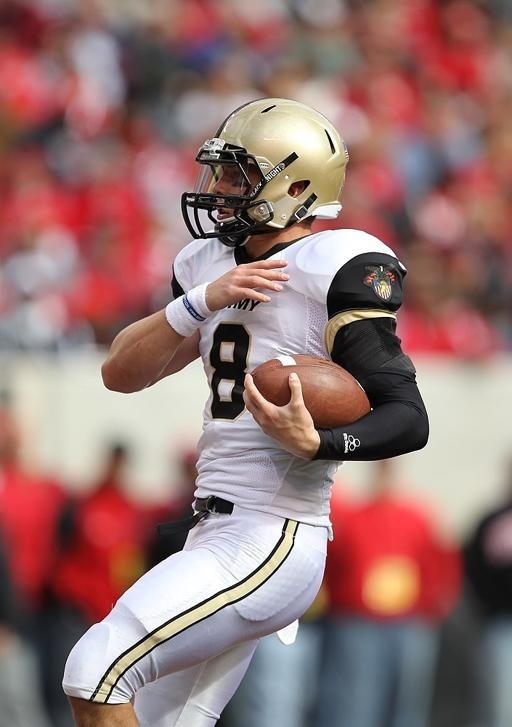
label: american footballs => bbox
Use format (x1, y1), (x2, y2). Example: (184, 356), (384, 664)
(250, 355), (371, 430)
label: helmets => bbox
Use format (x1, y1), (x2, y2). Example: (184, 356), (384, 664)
(179, 94), (350, 244)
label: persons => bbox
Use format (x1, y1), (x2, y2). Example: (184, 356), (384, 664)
(60, 92), (431, 724)
(0, 396), (510, 725)
(0, 0), (511, 358)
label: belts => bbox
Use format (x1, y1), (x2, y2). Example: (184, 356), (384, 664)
(182, 496), (236, 524)
